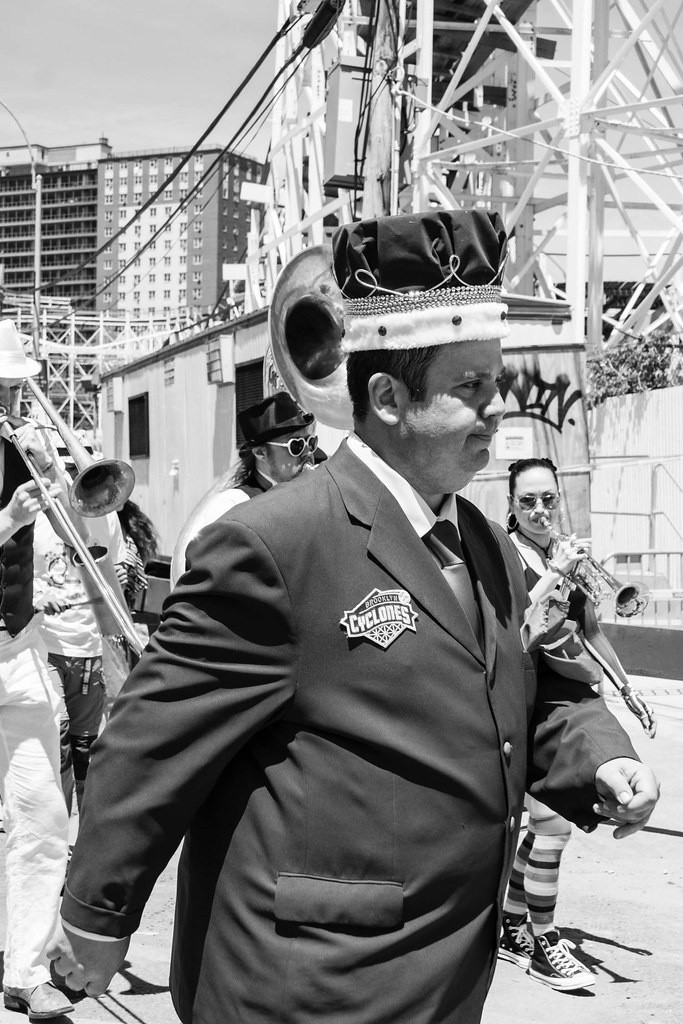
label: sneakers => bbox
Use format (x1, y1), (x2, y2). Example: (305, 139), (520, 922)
(526, 931), (595, 989)
(497, 910), (534, 968)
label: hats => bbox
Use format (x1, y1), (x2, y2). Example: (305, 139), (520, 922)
(50, 429), (104, 463)
(331, 210), (510, 352)
(0, 319), (43, 378)
(237, 391), (314, 450)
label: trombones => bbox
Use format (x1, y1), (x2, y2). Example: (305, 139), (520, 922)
(0, 376), (146, 659)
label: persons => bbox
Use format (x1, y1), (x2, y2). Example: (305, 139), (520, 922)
(32, 484), (124, 818)
(46, 212), (658, 1024)
(170, 391), (318, 595)
(497, 459), (657, 992)
(0, 318), (88, 1021)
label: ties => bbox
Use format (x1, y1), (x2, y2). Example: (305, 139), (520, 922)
(423, 520), (485, 658)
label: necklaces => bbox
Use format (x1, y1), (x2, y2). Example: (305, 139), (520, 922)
(517, 527), (552, 558)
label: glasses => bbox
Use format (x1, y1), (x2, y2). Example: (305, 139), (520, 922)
(511, 494), (561, 510)
(266, 435), (319, 457)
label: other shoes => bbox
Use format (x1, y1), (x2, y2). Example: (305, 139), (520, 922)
(3, 981), (75, 1018)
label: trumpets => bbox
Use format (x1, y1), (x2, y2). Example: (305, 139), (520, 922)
(539, 516), (650, 618)
